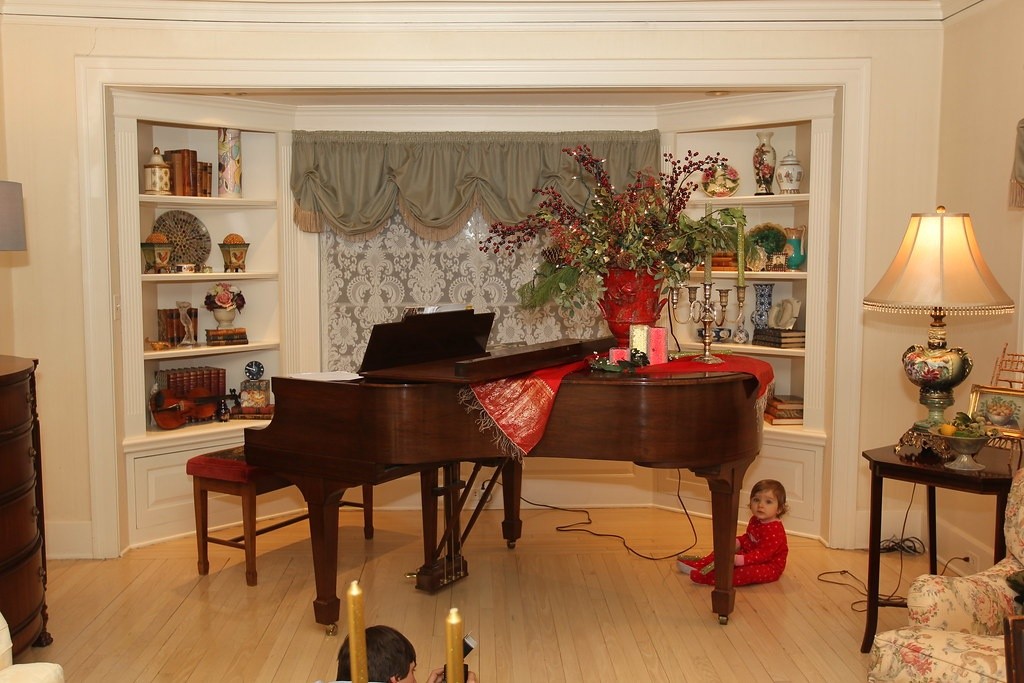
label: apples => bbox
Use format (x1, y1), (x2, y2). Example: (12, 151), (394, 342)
(940, 423), (955, 436)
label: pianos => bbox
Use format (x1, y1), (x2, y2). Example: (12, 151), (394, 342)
(241, 304), (769, 637)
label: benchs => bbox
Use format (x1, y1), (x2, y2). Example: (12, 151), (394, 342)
(187, 444), (375, 587)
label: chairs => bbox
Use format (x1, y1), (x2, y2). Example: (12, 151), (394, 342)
(0, 612), (63, 682)
(866, 468), (1024, 683)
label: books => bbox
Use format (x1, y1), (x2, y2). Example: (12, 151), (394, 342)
(154, 366), (226, 423)
(696, 250), (750, 270)
(205, 327), (249, 346)
(765, 394), (803, 426)
(157, 308), (198, 346)
(229, 403), (275, 420)
(752, 327), (806, 349)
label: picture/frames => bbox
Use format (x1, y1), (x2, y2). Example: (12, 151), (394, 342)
(966, 383), (1024, 440)
(768, 252), (788, 271)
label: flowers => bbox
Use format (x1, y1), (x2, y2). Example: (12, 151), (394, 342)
(590, 346), (652, 374)
(479, 141), (729, 311)
(200, 283), (246, 315)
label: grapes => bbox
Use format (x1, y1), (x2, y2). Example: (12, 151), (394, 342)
(962, 411), (986, 437)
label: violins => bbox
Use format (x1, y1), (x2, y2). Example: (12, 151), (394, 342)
(149, 387), (241, 431)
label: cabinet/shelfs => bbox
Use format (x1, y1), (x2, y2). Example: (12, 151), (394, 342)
(0, 355), (54, 656)
(115, 95), (301, 452)
(666, 89), (838, 434)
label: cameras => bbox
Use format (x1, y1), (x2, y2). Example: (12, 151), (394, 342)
(443, 631), (477, 683)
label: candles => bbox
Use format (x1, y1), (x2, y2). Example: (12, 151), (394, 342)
(445, 608), (465, 683)
(704, 203), (712, 283)
(608, 347), (631, 365)
(348, 579), (368, 683)
(737, 223), (744, 285)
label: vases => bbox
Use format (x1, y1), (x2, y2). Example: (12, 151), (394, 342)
(752, 131), (776, 196)
(750, 282), (776, 329)
(595, 266), (667, 350)
(213, 309), (237, 331)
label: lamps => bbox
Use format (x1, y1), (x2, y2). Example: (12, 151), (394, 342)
(862, 206), (1017, 460)
(0, 181), (27, 251)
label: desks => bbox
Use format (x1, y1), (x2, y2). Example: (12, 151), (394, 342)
(860, 443), (1022, 652)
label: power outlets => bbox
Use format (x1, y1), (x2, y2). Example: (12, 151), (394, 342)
(468, 489), (493, 500)
(967, 551), (981, 572)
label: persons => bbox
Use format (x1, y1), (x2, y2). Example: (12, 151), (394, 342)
(334, 625), (477, 683)
(675, 479), (789, 587)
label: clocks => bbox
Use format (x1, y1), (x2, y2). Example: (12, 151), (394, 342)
(244, 360), (264, 380)
